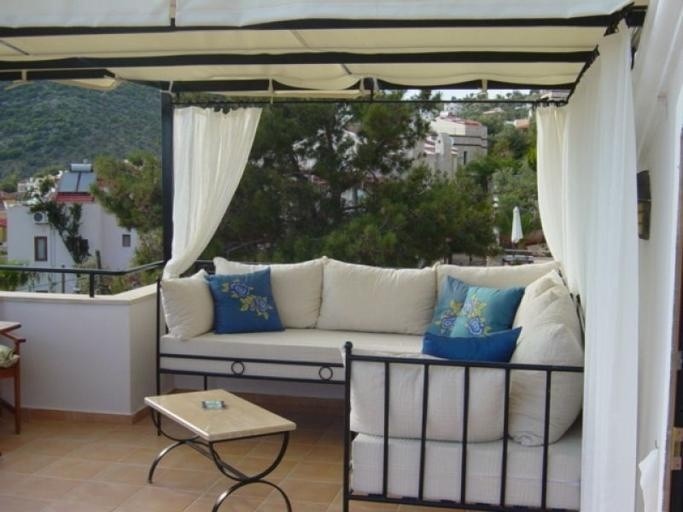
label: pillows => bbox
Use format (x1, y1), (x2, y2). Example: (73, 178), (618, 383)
(421, 329), (521, 367)
(342, 351), (513, 442)
(211, 252), (327, 332)
(433, 255), (559, 300)
(506, 267), (583, 446)
(425, 271), (528, 336)
(159, 269), (229, 338)
(202, 269), (285, 335)
(314, 251), (439, 341)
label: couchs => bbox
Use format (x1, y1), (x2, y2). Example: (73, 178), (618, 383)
(150, 258), (597, 511)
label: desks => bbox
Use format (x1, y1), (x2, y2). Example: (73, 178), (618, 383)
(1, 318), (28, 436)
